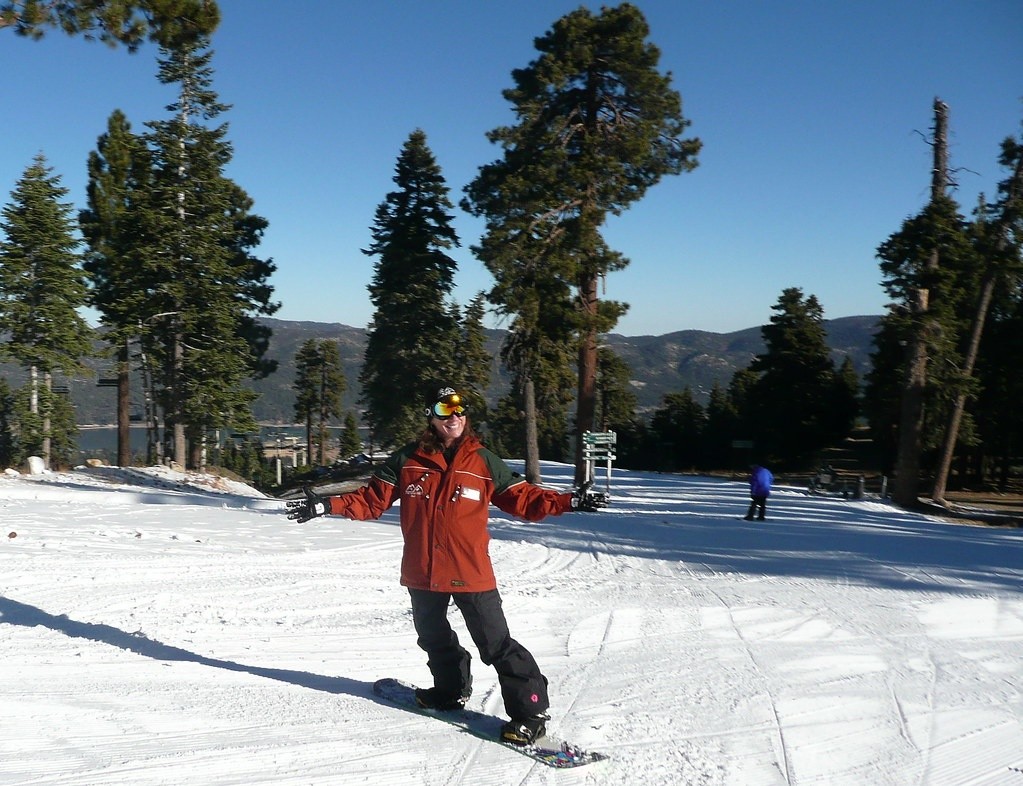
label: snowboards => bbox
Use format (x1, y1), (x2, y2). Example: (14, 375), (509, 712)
(374, 678), (610, 769)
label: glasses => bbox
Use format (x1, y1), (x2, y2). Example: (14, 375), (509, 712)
(424, 393), (470, 420)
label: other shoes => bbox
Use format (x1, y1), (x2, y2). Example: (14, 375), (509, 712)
(756, 516), (765, 520)
(499, 713), (551, 747)
(743, 516), (753, 521)
(414, 686), (466, 709)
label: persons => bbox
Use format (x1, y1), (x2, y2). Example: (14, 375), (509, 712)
(284, 378), (612, 747)
(744, 464), (774, 521)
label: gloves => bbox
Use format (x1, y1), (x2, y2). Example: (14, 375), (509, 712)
(570, 479), (611, 512)
(285, 485), (332, 524)
